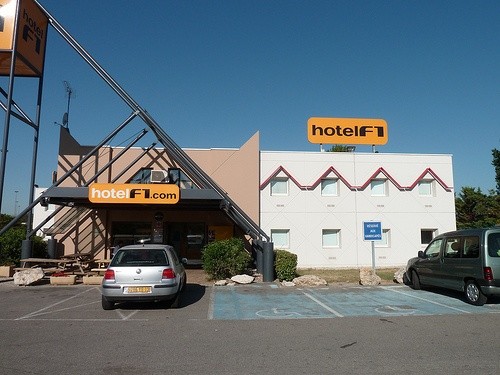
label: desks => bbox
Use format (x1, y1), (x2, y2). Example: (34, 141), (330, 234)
(59, 253), (92, 270)
(95, 259), (111, 275)
(20, 258), (84, 275)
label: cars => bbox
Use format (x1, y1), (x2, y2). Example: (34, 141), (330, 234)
(101, 243), (188, 311)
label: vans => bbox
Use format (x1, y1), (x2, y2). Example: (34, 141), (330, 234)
(407, 228), (499, 307)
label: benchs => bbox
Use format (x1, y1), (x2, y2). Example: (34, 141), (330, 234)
(13, 258), (108, 279)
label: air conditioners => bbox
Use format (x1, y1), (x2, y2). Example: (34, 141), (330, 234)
(151, 170), (170, 183)
